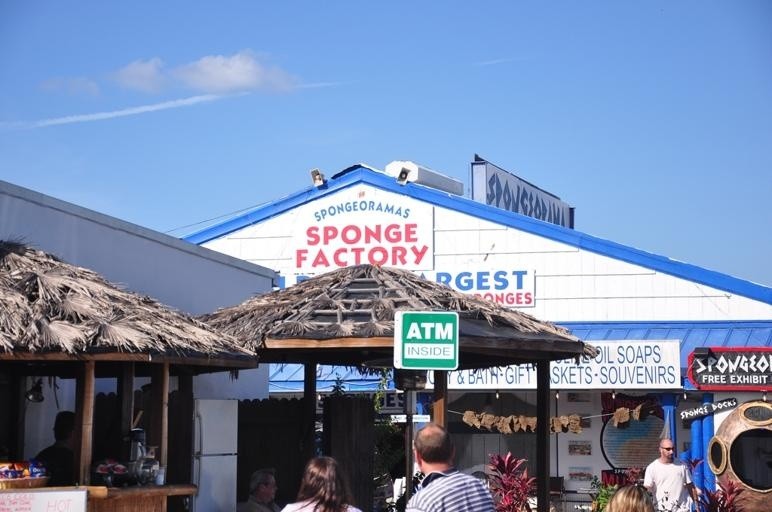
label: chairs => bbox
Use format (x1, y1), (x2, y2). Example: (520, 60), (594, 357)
(549, 477), (564, 503)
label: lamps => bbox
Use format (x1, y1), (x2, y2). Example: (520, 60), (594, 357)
(317, 392), (322, 401)
(761, 391), (767, 402)
(24, 377), (45, 403)
(495, 389), (688, 401)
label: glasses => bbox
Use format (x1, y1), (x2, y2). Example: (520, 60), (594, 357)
(661, 446), (675, 451)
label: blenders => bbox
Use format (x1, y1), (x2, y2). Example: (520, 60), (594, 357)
(128, 428), (148, 483)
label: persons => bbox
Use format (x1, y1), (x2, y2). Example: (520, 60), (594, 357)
(604, 485), (656, 512)
(237, 467), (284, 511)
(642, 436), (700, 511)
(279, 455), (366, 512)
(32, 407), (80, 487)
(403, 422), (495, 512)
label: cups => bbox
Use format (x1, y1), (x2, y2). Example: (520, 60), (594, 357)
(153, 464), (168, 487)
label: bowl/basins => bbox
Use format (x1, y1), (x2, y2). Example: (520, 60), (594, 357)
(96, 472), (127, 488)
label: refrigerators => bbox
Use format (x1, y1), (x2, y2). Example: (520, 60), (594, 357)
(189, 398), (238, 512)
